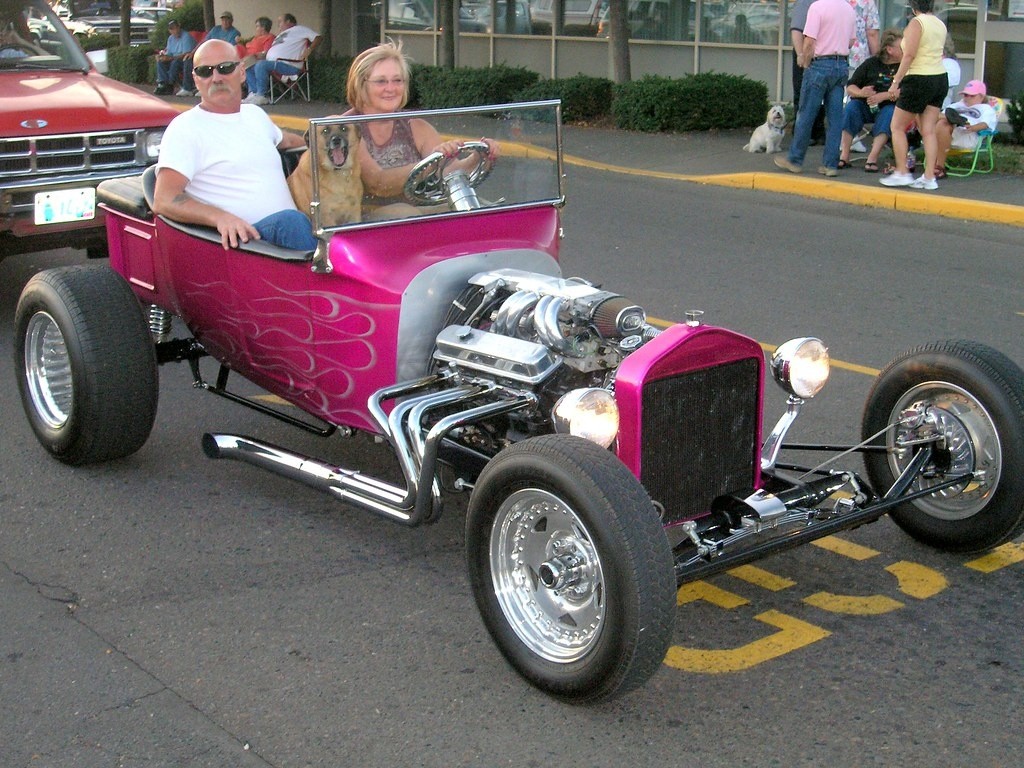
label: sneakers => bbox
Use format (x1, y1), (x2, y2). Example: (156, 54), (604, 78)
(879, 173), (913, 186)
(909, 173), (939, 190)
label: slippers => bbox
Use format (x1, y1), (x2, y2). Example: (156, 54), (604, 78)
(837, 160), (852, 168)
(864, 163), (878, 173)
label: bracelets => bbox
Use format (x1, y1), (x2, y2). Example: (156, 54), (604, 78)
(893, 80), (896, 83)
(308, 47), (311, 50)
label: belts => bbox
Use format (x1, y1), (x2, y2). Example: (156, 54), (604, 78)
(812, 55), (845, 61)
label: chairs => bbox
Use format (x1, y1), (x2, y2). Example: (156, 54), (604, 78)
(174, 31), (311, 105)
(849, 96), (1004, 177)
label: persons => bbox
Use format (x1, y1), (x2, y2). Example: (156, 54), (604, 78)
(879, 0), (948, 191)
(840, 0), (880, 153)
(154, 20), (196, 95)
(176, 11), (242, 97)
(774, 0), (856, 177)
(0, 12), (50, 58)
(242, 14), (323, 105)
(789, 0), (825, 146)
(933, 80), (997, 179)
(882, 34), (962, 174)
(152, 39), (319, 250)
(237, 17), (275, 58)
(342, 44), (497, 217)
(840, 29), (903, 172)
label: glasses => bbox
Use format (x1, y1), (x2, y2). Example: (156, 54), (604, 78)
(964, 94), (976, 97)
(191, 62), (240, 77)
(0, 21), (17, 31)
(364, 77), (405, 86)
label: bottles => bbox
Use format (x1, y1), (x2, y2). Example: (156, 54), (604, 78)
(866, 97), (880, 113)
(906, 146), (916, 173)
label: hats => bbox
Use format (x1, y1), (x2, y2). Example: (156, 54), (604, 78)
(220, 11), (232, 18)
(958, 81), (986, 96)
(168, 20), (177, 27)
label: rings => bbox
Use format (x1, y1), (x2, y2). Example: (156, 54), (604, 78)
(891, 94), (893, 97)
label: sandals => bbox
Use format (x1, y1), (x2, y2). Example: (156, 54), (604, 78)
(945, 108), (967, 126)
(934, 165), (946, 179)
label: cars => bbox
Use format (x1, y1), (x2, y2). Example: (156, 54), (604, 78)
(13, 100), (1024, 709)
(0, 0), (183, 259)
(26, 0), (175, 56)
(385, 0), (796, 47)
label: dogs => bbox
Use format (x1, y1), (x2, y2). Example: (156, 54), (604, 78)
(287, 115), (363, 229)
(742, 105), (786, 154)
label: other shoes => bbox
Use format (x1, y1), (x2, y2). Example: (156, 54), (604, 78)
(774, 156), (802, 173)
(195, 91), (201, 97)
(241, 92), (258, 103)
(154, 83), (174, 95)
(809, 139), (817, 146)
(850, 141), (867, 153)
(818, 139), (825, 145)
(175, 88), (194, 96)
(249, 95), (269, 105)
(817, 166), (838, 177)
